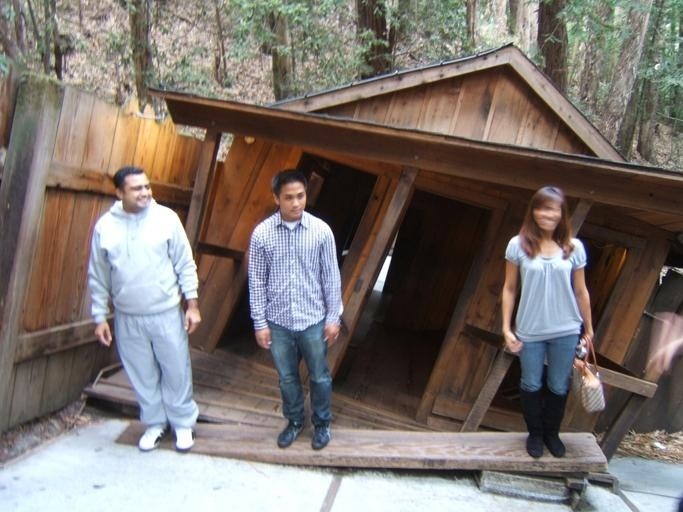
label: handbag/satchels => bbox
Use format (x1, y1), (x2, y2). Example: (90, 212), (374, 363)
(570, 335), (606, 412)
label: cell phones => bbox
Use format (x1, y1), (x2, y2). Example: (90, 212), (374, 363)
(578, 345), (586, 359)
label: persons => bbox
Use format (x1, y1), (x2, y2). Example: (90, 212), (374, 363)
(86, 166), (204, 452)
(248, 168), (345, 449)
(498, 186), (597, 459)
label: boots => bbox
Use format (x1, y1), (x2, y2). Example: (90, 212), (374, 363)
(517, 383), (545, 459)
(543, 381), (569, 457)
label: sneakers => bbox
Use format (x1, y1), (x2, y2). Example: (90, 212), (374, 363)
(310, 420), (331, 451)
(173, 425), (196, 450)
(137, 419), (168, 452)
(275, 417), (305, 449)
